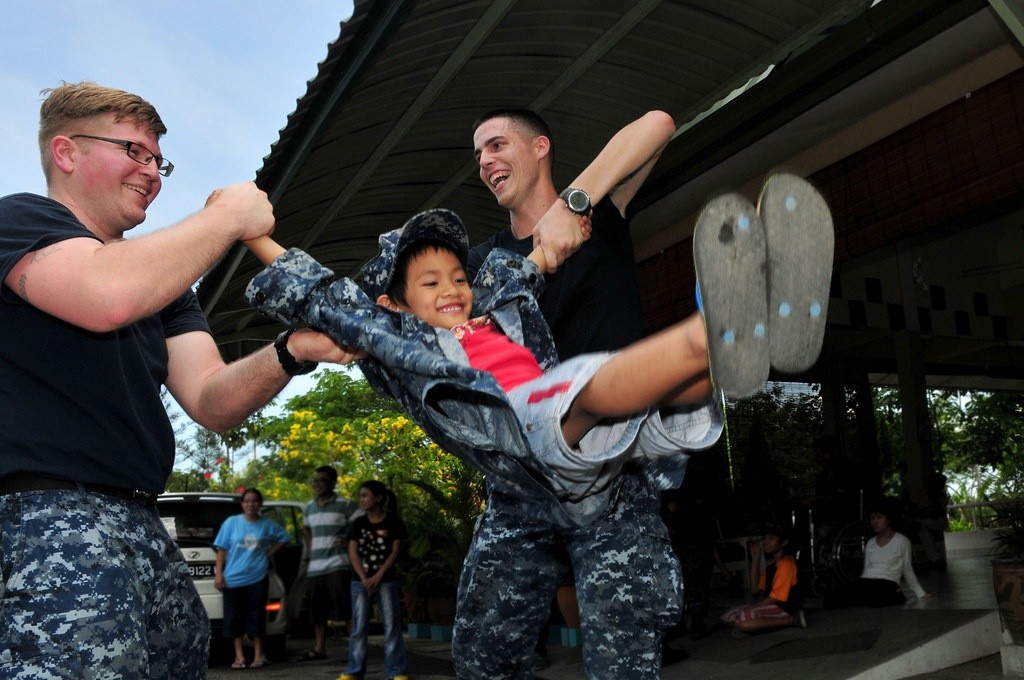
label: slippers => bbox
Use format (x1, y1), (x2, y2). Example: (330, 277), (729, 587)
(232, 662), (246, 669)
(249, 659), (271, 669)
(341, 655), (348, 663)
(296, 647), (327, 662)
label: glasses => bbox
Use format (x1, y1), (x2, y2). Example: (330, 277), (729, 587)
(69, 134), (174, 177)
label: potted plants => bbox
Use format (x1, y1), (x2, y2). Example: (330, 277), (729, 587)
(394, 465), (580, 627)
(981, 480), (1024, 645)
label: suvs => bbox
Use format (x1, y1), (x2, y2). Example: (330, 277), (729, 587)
(154, 491), (317, 662)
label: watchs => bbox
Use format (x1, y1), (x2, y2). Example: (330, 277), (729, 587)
(559, 188), (592, 216)
(275, 327), (319, 376)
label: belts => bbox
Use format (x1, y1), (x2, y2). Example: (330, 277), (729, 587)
(0, 474), (158, 508)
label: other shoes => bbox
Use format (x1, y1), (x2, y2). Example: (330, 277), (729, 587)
(561, 624), (569, 647)
(568, 628), (581, 648)
(792, 611), (807, 628)
(692, 193), (771, 400)
(336, 672), (356, 680)
(691, 623), (706, 640)
(731, 628), (750, 639)
(534, 653), (549, 669)
(661, 640), (688, 666)
(755, 172), (836, 373)
(394, 675), (408, 680)
(665, 624), (688, 639)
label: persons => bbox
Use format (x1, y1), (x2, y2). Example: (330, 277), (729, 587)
(214, 488), (291, 668)
(666, 435), (930, 640)
(1, 80), (364, 680)
(451, 108), (687, 680)
(300, 466), (409, 680)
(204, 172), (835, 528)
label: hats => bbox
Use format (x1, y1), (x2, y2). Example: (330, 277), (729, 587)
(360, 209), (469, 304)
